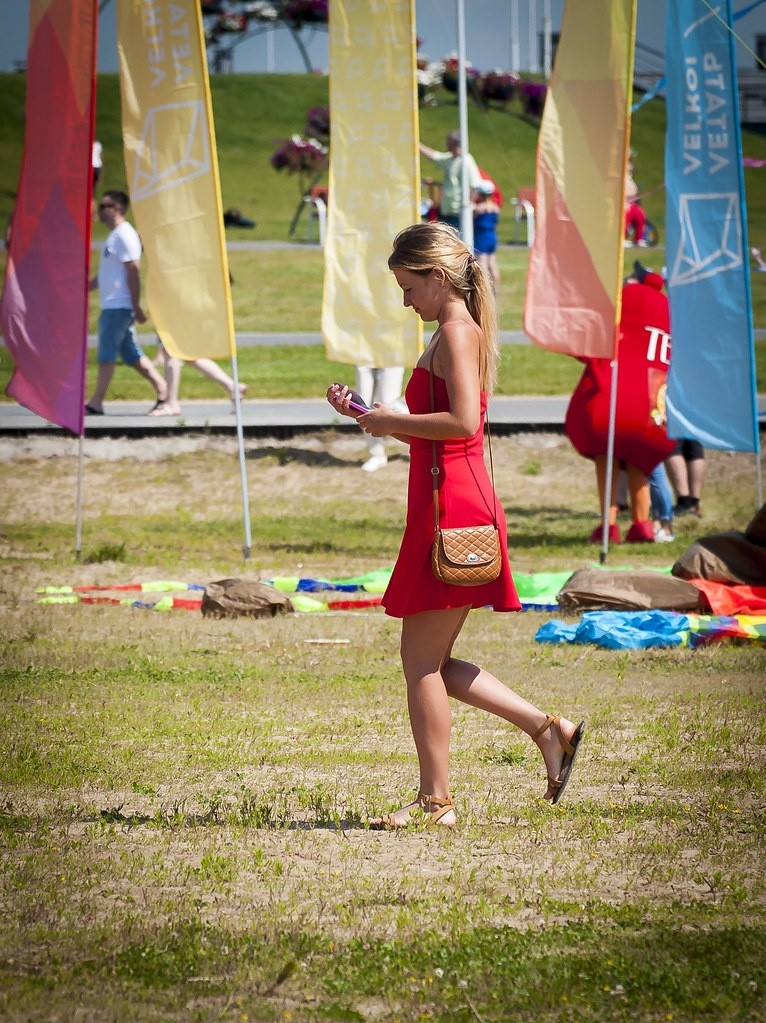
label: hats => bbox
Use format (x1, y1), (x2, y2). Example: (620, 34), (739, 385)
(478, 179), (494, 197)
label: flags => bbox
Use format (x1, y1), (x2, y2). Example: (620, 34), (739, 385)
(320, 1), (421, 371)
(117, 0), (236, 362)
(664, 0), (760, 457)
(0, 0), (99, 435)
(522, 0), (633, 359)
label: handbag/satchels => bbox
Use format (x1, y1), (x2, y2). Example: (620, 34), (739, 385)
(433, 525), (501, 586)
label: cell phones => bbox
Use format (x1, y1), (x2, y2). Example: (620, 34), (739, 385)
(333, 392), (375, 413)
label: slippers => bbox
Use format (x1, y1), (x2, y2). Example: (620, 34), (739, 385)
(84, 405), (105, 416)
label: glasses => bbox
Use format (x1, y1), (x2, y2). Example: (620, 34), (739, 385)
(99, 203), (116, 211)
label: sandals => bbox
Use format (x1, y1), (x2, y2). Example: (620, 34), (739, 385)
(532, 714), (585, 805)
(370, 792), (457, 830)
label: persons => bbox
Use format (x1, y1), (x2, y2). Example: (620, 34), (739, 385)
(477, 166), (503, 207)
(623, 163), (648, 249)
(475, 180), (500, 293)
(416, 36), (429, 101)
(327, 223), (586, 824)
(564, 256), (706, 545)
(356, 366), (410, 472)
(750, 245), (766, 272)
(422, 182), (444, 224)
(82, 189), (167, 415)
(420, 128), (481, 231)
(92, 139), (103, 192)
(150, 347), (247, 416)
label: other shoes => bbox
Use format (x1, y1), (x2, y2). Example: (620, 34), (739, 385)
(654, 528), (674, 544)
(150, 402), (181, 417)
(231, 383), (248, 413)
(672, 496), (699, 517)
